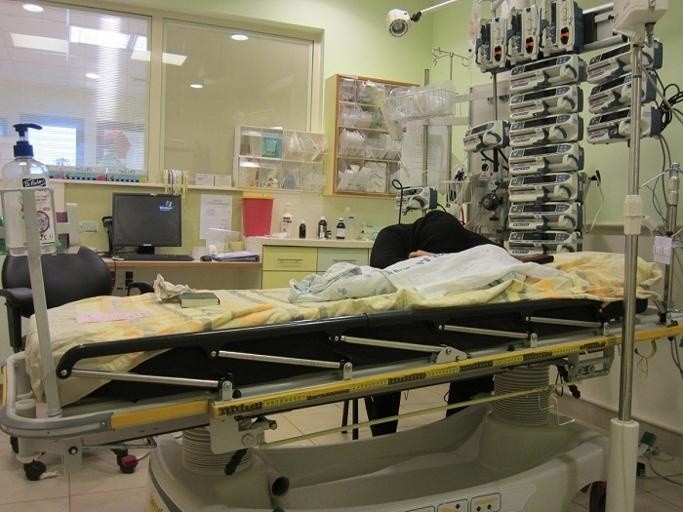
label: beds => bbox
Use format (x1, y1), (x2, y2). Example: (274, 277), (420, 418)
(18, 251), (656, 512)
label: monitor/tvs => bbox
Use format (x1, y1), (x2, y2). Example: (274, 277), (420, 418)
(112, 192), (182, 254)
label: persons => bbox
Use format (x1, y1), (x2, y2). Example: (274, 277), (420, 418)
(361, 210), (507, 439)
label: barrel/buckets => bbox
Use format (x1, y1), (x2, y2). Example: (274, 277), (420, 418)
(240, 193), (275, 236)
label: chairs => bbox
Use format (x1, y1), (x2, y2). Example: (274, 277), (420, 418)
(0, 243), (152, 353)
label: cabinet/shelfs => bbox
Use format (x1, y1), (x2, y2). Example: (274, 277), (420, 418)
(231, 74), (419, 200)
(263, 246), (369, 290)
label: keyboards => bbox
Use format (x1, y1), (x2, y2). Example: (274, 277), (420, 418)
(119, 254), (193, 261)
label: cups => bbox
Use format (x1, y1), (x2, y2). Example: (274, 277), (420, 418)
(16, 399), (36, 417)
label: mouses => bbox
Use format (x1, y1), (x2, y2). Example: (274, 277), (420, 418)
(200, 255), (211, 261)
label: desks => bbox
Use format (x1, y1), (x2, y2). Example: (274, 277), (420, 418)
(98, 254), (262, 298)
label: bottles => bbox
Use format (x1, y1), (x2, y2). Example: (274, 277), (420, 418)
(282, 214), (354, 240)
